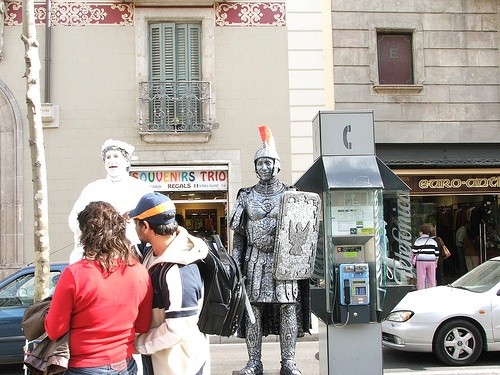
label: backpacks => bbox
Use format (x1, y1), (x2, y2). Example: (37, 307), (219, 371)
(141, 234), (246, 338)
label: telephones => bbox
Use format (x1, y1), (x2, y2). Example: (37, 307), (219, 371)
(331, 263), (370, 329)
(310, 220), (324, 284)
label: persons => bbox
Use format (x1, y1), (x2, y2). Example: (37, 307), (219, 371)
(456, 220), (470, 278)
(122, 192), (211, 375)
(424, 223), (445, 286)
(411, 224), (440, 289)
(44, 201), (153, 375)
(463, 224), (479, 272)
(228, 125), (313, 375)
(68, 138), (155, 269)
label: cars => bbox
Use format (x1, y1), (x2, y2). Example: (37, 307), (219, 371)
(380, 255), (500, 367)
(0, 263), (71, 364)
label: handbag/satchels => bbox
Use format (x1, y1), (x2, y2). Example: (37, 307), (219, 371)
(412, 255), (417, 267)
(438, 237), (451, 259)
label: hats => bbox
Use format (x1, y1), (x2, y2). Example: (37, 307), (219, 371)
(123, 192), (175, 220)
